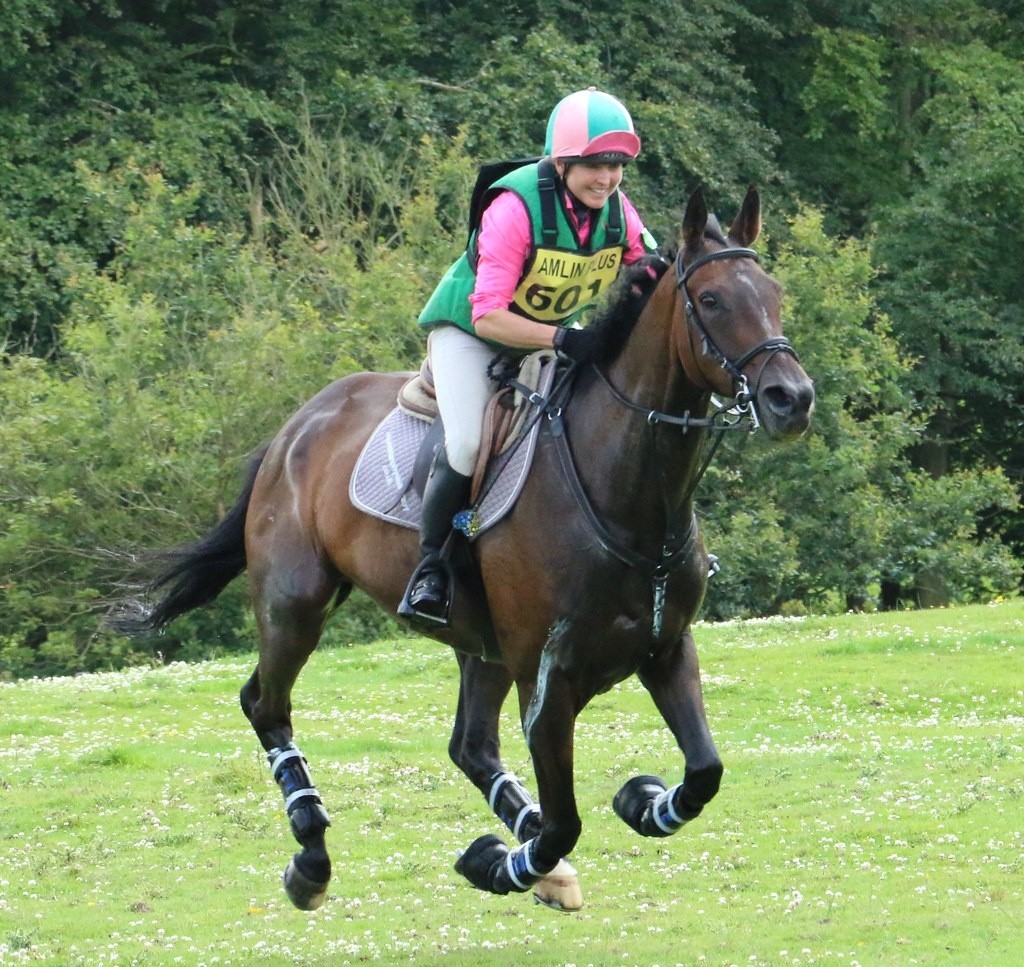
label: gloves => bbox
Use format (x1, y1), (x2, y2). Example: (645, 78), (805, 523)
(552, 325), (605, 366)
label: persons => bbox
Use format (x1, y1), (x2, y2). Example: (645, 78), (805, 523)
(408, 86), (670, 621)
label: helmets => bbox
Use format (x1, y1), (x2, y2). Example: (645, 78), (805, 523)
(543, 85), (641, 165)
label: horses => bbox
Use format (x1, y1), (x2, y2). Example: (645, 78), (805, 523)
(81, 181), (816, 910)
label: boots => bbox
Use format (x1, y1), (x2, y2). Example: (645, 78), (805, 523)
(408, 443), (475, 618)
